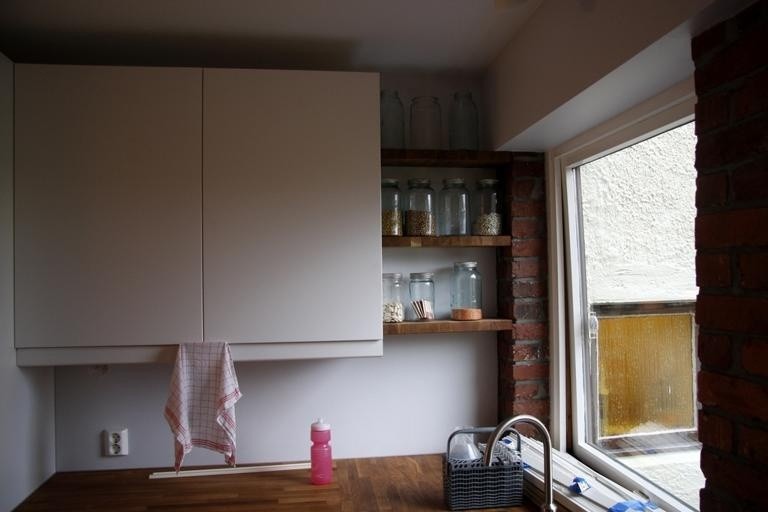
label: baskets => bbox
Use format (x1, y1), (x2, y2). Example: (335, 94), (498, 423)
(441, 423), (530, 511)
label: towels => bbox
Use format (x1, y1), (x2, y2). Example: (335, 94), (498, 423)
(162, 341), (243, 475)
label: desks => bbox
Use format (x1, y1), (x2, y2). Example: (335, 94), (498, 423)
(10, 454), (447, 512)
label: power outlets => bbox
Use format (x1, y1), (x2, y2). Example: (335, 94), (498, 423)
(104, 429), (128, 455)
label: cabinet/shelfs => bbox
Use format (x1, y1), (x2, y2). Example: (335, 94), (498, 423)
(14, 63), (386, 369)
(381, 147), (512, 334)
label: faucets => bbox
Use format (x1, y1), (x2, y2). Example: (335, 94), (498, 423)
(484, 415), (559, 512)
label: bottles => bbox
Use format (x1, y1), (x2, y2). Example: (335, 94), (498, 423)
(310, 419), (333, 486)
(381, 86), (503, 323)
